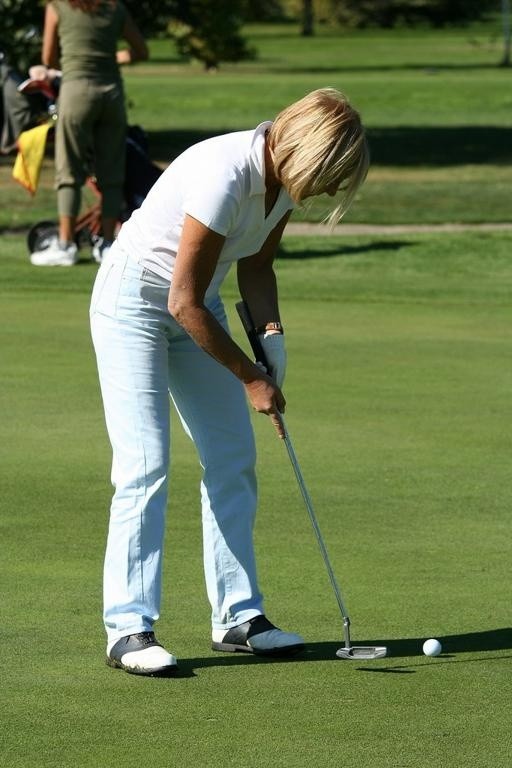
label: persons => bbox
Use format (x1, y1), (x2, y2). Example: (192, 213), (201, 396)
(27, 1), (131, 268)
(88, 81), (374, 679)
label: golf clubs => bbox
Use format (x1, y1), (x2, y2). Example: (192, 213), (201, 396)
(233, 302), (391, 659)
(17, 63), (63, 99)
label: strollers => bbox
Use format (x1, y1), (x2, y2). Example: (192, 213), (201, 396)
(15, 55), (166, 257)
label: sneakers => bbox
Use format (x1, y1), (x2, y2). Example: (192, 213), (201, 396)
(211, 616), (307, 656)
(29, 237), (80, 267)
(105, 632), (179, 674)
(93, 236), (113, 263)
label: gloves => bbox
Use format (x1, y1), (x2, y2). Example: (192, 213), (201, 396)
(253, 332), (287, 389)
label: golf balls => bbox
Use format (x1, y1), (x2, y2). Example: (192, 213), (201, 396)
(423, 639), (442, 657)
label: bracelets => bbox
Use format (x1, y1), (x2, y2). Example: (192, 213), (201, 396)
(253, 320), (284, 334)
